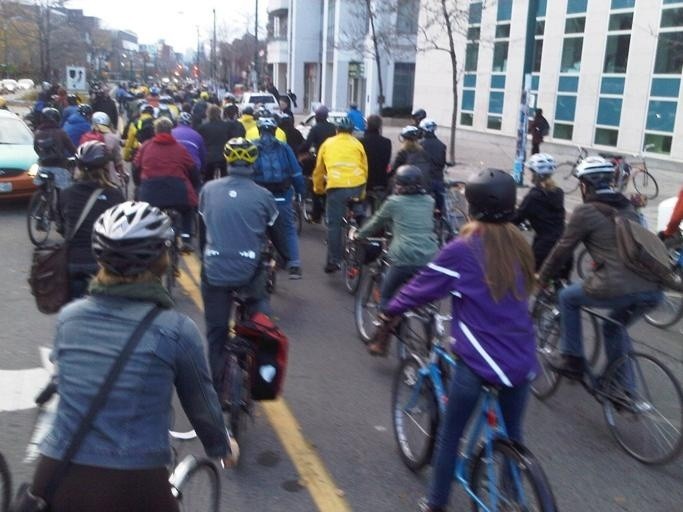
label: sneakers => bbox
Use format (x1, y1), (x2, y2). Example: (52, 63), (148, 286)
(415, 494), (445, 511)
(365, 322), (394, 359)
(611, 388), (654, 418)
(541, 348), (589, 380)
(325, 259), (344, 275)
(284, 263), (306, 281)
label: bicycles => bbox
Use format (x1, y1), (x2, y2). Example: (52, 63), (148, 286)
(221, 245), (282, 445)
(392, 303), (558, 512)
(160, 208), (181, 296)
(353, 232), (452, 368)
(578, 248), (595, 281)
(433, 178), (470, 250)
(26, 157), (78, 246)
(526, 266), (683, 465)
(323, 197), (365, 296)
(554, 142), (588, 193)
(265, 179), (322, 267)
(35, 375), (227, 511)
(643, 227), (683, 329)
(599, 142), (662, 200)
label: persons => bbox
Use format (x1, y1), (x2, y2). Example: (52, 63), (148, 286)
(374, 125), (434, 198)
(222, 93), (243, 117)
(527, 108), (550, 155)
(533, 155), (665, 414)
(92, 89), (117, 129)
(250, 117), (304, 281)
(54, 139), (125, 300)
(197, 136), (291, 412)
(170, 111), (208, 190)
(277, 112), (307, 156)
(377, 166), (558, 512)
(30, 75), (73, 112)
(657, 189), (683, 273)
(357, 116), (391, 259)
(30, 200), (241, 512)
(253, 102), (272, 121)
(77, 111), (130, 186)
(123, 104), (158, 163)
(131, 116), (201, 252)
(345, 103), (368, 139)
(245, 107), (287, 144)
(238, 106), (253, 129)
(514, 153), (575, 294)
(418, 121), (454, 243)
(33, 106), (79, 236)
(222, 105), (246, 138)
(311, 117), (368, 274)
(197, 104), (232, 180)
(62, 104), (93, 146)
(348, 164), (448, 382)
(115, 77), (220, 115)
(411, 108), (426, 128)
(294, 104), (338, 224)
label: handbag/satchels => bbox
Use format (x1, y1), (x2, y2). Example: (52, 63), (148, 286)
(594, 200), (675, 286)
(6, 481), (47, 509)
(26, 233), (70, 317)
(232, 311), (287, 403)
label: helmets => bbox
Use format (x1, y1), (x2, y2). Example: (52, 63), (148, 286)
(201, 91), (209, 101)
(148, 86), (161, 95)
(465, 167), (516, 217)
(223, 92), (236, 101)
(139, 104), (155, 115)
(399, 125), (421, 141)
(572, 154), (615, 177)
(349, 101), (357, 110)
(89, 109), (112, 127)
(73, 141), (114, 172)
(89, 200), (175, 279)
(366, 115), (382, 130)
(313, 104), (328, 120)
(176, 112), (193, 126)
(523, 153), (557, 176)
(151, 115), (175, 130)
(66, 93), (77, 100)
(222, 103), (240, 114)
(334, 117), (354, 131)
(38, 107), (62, 127)
(49, 94), (63, 106)
(222, 136), (260, 166)
(256, 116), (278, 129)
(77, 103), (93, 114)
(418, 117), (437, 133)
(394, 164), (424, 186)
(414, 108), (426, 120)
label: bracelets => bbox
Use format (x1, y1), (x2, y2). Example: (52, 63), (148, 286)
(353, 230), (360, 240)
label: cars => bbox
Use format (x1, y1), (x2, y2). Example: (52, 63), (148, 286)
(240, 92), (281, 115)
(294, 112), (367, 142)
(0, 109), (39, 198)
